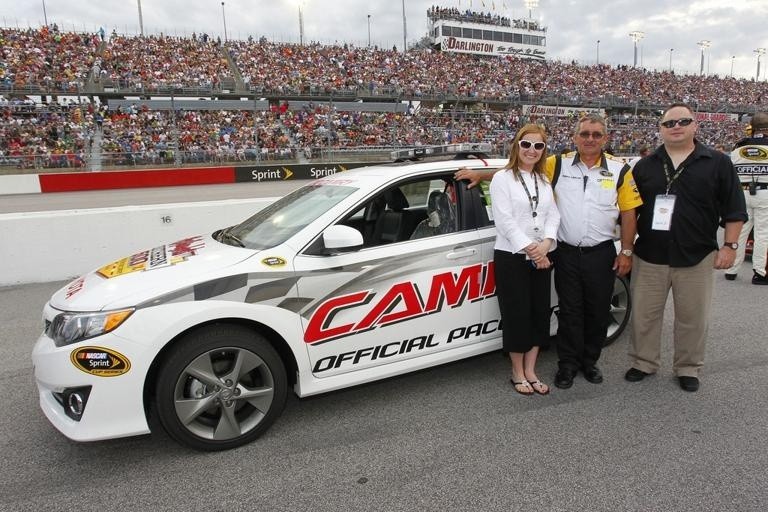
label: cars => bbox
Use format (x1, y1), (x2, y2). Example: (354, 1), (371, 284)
(31, 142), (632, 452)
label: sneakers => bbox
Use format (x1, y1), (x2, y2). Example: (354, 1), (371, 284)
(725, 274), (737, 280)
(752, 269), (768, 285)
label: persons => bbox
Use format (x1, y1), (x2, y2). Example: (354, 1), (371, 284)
(489, 123), (561, 396)
(453, 113), (644, 389)
(0, 6), (768, 168)
(723, 111), (768, 285)
(616, 102), (748, 392)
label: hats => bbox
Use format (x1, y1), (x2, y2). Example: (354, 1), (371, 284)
(752, 112), (768, 130)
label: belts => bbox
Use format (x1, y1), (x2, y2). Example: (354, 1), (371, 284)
(741, 185), (768, 190)
(559, 240), (614, 252)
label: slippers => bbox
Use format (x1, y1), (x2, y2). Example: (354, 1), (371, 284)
(511, 378), (549, 395)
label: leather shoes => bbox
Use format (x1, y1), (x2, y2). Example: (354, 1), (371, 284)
(625, 368), (648, 381)
(579, 365), (603, 383)
(555, 367), (576, 388)
(680, 376), (699, 392)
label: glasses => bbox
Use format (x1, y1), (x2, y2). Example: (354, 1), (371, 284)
(661, 118), (692, 127)
(519, 140), (545, 151)
(580, 131), (603, 139)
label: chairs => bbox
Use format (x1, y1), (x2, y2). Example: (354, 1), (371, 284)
(369, 187), (409, 247)
(409, 189), (455, 240)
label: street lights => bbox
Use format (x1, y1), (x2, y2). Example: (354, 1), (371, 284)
(219, 1), (371, 46)
(590, 22), (763, 74)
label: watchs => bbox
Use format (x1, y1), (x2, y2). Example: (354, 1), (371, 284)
(724, 242), (739, 250)
(620, 249), (634, 257)
